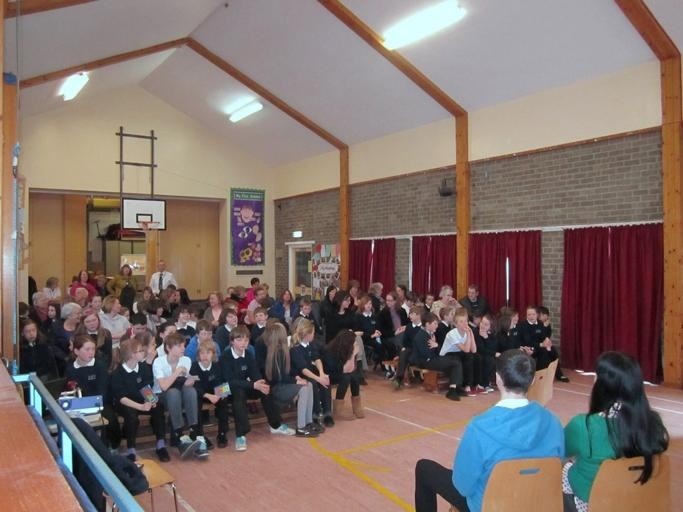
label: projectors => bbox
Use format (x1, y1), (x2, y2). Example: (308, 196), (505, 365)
(59, 394), (104, 413)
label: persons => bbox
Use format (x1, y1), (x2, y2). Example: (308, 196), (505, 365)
(318, 278), (560, 402)
(19, 258), (365, 460)
(561, 349), (670, 512)
(415, 350), (566, 510)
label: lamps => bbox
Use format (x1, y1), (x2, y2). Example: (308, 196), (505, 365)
(437, 176), (455, 198)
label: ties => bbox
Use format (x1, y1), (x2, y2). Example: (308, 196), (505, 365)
(159, 274), (163, 289)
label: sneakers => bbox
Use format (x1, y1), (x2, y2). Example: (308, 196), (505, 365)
(156, 396), (365, 462)
(386, 370), (424, 388)
(447, 385), (494, 400)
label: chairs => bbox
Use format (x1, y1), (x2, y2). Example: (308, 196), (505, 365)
(447, 455), (569, 512)
(100, 457), (179, 512)
(586, 456), (671, 512)
